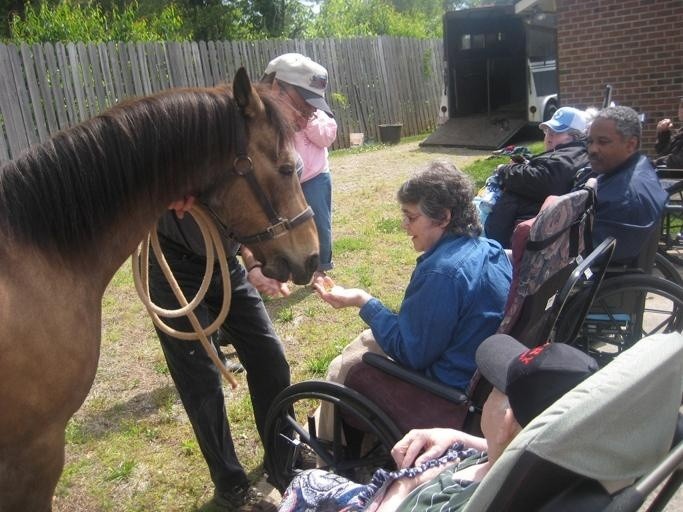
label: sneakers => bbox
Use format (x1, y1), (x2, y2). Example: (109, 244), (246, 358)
(213, 482), (282, 512)
(264, 444), (315, 475)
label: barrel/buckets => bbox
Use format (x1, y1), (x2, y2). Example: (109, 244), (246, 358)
(350, 133), (364, 148)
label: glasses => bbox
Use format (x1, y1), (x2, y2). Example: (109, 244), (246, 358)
(273, 77), (317, 125)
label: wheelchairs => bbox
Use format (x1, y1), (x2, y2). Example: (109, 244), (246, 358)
(265, 189), (619, 499)
(504, 158), (682, 369)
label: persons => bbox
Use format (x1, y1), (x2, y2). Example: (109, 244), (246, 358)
(292, 106), (337, 288)
(470, 104), (590, 248)
(277, 331), (601, 511)
(136, 50), (335, 512)
(585, 104), (669, 266)
(311, 156), (513, 467)
(650, 92), (682, 169)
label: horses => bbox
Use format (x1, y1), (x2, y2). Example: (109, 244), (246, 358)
(0, 63), (325, 512)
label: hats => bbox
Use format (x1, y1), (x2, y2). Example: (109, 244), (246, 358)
(263, 53), (335, 118)
(538, 107), (588, 134)
(474, 334), (599, 428)
